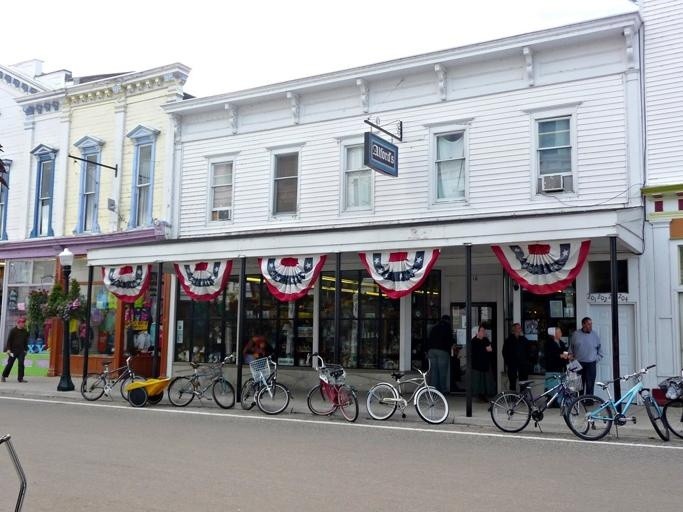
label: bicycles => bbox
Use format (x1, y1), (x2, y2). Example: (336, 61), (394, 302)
(236, 351), (297, 416)
(301, 350), (361, 424)
(662, 392), (682, 441)
(563, 363), (670, 444)
(363, 354), (449, 425)
(166, 351), (242, 408)
(77, 349), (153, 401)
(485, 364), (593, 436)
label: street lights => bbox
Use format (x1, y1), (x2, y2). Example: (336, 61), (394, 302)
(56, 247), (76, 393)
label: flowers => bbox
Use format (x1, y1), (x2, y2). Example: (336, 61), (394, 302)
(39, 278), (88, 322)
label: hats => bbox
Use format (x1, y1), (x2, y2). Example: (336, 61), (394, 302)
(17, 318), (26, 324)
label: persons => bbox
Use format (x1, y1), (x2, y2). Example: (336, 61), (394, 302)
(567, 315), (604, 405)
(501, 322), (533, 405)
(543, 325), (568, 413)
(242, 326), (268, 364)
(426, 312), (454, 395)
(0, 316), (27, 382)
(468, 324), (492, 403)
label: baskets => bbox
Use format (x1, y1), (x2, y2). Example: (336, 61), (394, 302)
(249, 357), (271, 382)
(563, 375), (583, 394)
(658, 376), (681, 397)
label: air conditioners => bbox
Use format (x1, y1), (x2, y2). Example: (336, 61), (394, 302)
(541, 175), (564, 192)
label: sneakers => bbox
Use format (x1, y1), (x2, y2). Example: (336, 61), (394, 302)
(1, 377), (6, 382)
(19, 379), (28, 383)
